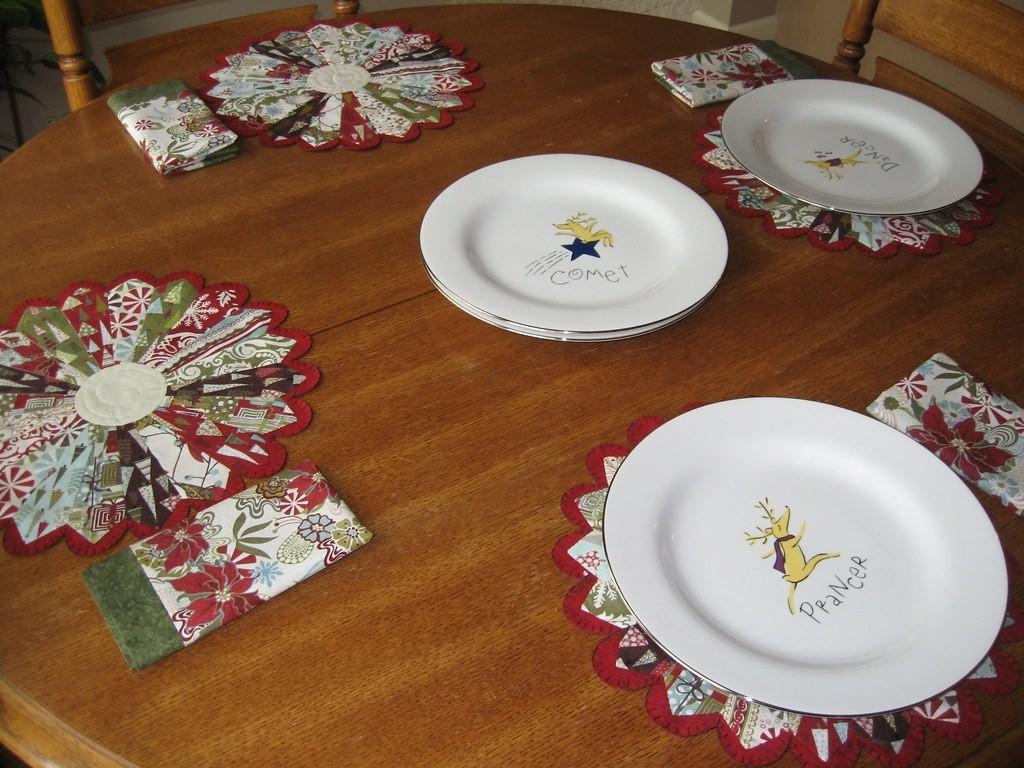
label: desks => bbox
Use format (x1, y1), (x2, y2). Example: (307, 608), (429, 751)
(0, 3), (1024, 768)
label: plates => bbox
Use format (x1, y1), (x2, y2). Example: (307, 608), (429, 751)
(419, 155), (728, 342)
(720, 79), (984, 217)
(604, 397), (1007, 719)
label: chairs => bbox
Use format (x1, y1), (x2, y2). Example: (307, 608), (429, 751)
(832, 0), (1024, 173)
(42, 0), (361, 112)
